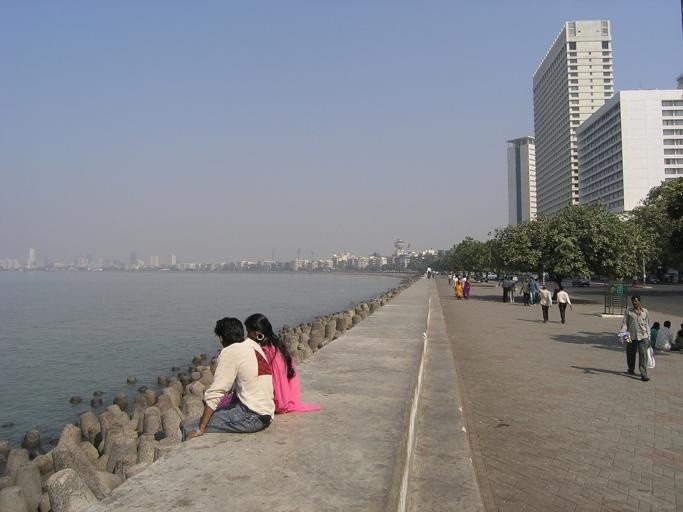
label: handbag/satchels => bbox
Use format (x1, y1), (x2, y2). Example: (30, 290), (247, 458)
(645, 344), (655, 369)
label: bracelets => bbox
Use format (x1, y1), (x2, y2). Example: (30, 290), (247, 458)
(194, 426), (202, 435)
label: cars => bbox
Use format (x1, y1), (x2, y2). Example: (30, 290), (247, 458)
(457, 271), (550, 283)
(571, 275), (590, 287)
(642, 274), (660, 284)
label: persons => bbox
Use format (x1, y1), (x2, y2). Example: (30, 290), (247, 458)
(538, 285), (553, 322)
(181, 317), (276, 442)
(650, 322), (660, 349)
(619, 295), (652, 381)
(557, 286), (573, 324)
(655, 320), (680, 351)
(674, 323), (683, 348)
(425, 266), (540, 307)
(217, 313), (321, 415)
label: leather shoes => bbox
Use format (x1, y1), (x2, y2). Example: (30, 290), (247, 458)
(641, 376), (650, 381)
(629, 371), (634, 375)
